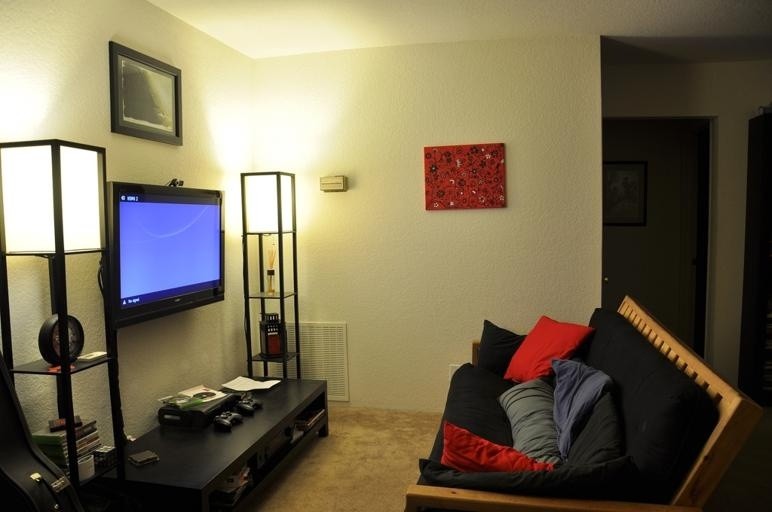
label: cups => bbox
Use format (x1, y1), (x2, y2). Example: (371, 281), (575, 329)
(77, 454), (95, 482)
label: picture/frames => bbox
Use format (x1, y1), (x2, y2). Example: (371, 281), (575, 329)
(110, 41), (183, 146)
(604, 161), (647, 227)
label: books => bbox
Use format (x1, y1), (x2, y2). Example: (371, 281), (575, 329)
(292, 404), (326, 430)
(26, 417), (100, 464)
(211, 462), (251, 503)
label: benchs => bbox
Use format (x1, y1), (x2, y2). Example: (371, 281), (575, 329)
(404, 296), (764, 512)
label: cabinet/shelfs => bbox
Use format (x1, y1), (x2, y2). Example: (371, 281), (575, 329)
(100, 376), (328, 511)
(241, 171), (300, 381)
(1, 139), (129, 511)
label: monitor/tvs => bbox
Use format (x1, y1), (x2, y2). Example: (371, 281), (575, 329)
(106, 181), (226, 330)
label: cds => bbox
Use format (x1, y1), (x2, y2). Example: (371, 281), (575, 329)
(193, 392), (216, 400)
